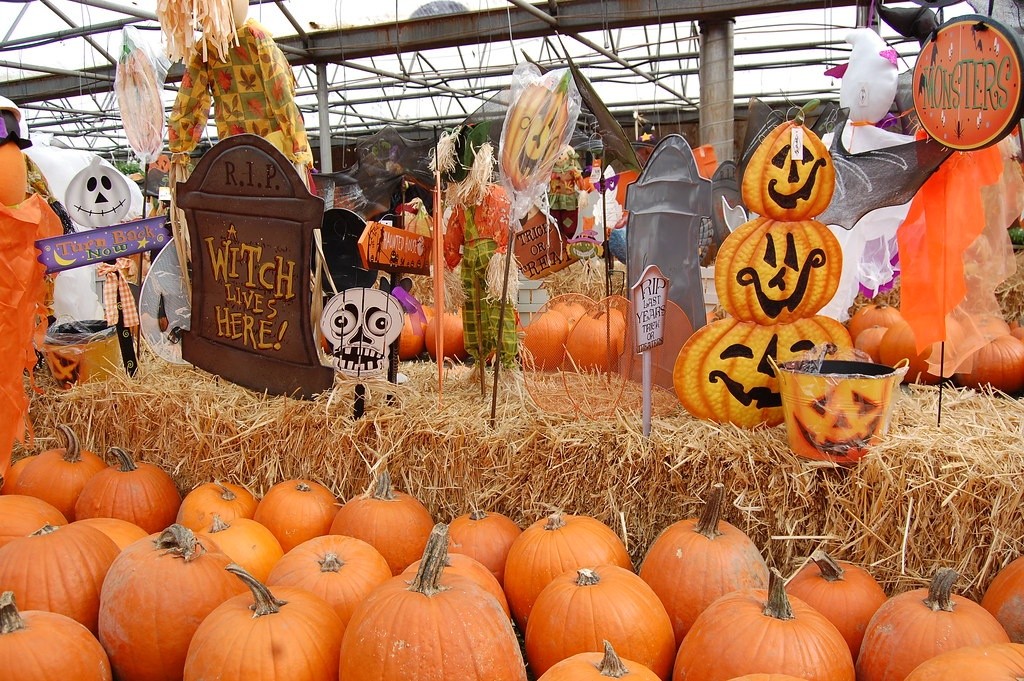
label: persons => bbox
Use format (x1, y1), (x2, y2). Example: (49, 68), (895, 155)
(155, 0), (339, 367)
(546, 144), (584, 244)
(426, 120), (521, 371)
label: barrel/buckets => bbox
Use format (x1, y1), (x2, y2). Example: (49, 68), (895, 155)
(38, 317), (118, 390)
(767, 354), (909, 462)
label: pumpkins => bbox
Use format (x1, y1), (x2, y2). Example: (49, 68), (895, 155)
(0, 424), (1024, 681)
(397, 298), (628, 375)
(848, 303), (1024, 398)
